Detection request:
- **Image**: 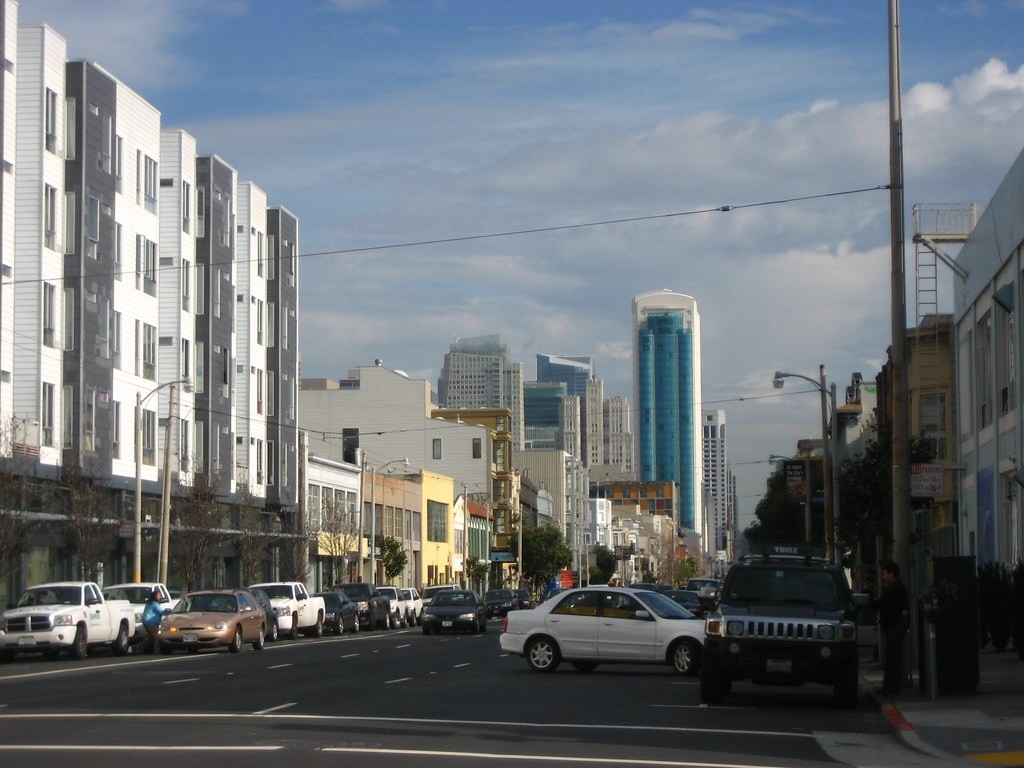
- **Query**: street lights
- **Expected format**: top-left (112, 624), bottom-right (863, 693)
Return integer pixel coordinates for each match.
top-left (773, 369), bottom-right (843, 569)
top-left (132, 376), bottom-right (196, 603)
top-left (768, 451), bottom-right (813, 561)
top-left (605, 516), bottom-right (632, 548)
top-left (368, 457), bottom-right (412, 585)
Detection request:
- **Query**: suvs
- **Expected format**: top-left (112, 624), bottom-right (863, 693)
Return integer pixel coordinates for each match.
top-left (156, 589), bottom-right (267, 656)
top-left (375, 586), bottom-right (410, 629)
top-left (700, 550), bottom-right (872, 703)
top-left (401, 587), bottom-right (422, 627)
top-left (328, 581), bottom-right (392, 632)
top-left (420, 584), bottom-right (469, 605)
top-left (422, 589), bottom-right (487, 636)
top-left (234, 588), bottom-right (279, 642)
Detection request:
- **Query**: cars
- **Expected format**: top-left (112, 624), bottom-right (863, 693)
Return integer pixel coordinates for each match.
top-left (500, 587), bottom-right (707, 674)
top-left (482, 577), bottom-right (724, 618)
top-left (309, 592), bottom-right (360, 636)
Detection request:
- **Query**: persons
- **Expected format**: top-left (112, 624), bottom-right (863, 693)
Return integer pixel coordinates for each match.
top-left (874, 562), bottom-right (913, 694)
top-left (141, 591), bottom-right (168, 655)
top-left (860, 575), bottom-right (876, 596)
top-left (461, 594), bottom-right (470, 603)
top-left (512, 595), bottom-right (521, 610)
top-left (224, 600), bottom-right (237, 613)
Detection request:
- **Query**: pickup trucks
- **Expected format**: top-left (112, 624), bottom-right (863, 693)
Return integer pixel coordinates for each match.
top-left (248, 580), bottom-right (326, 641)
top-left (101, 582), bottom-right (183, 643)
top-left (0, 581), bottom-right (137, 661)
